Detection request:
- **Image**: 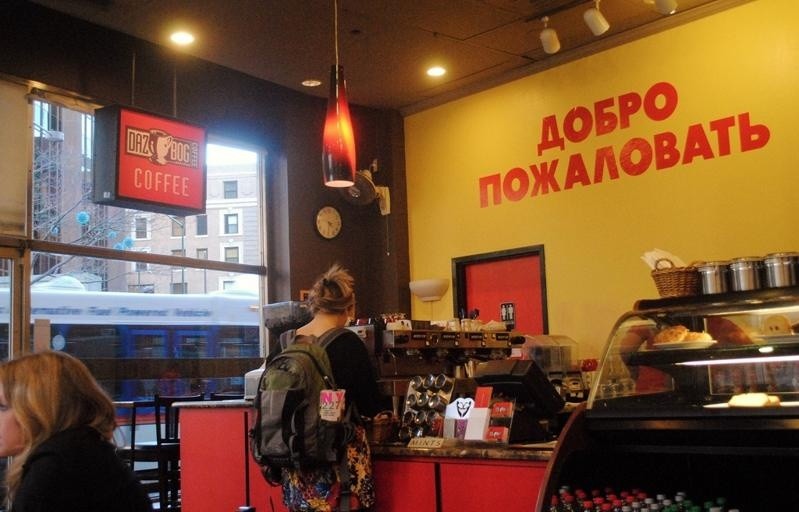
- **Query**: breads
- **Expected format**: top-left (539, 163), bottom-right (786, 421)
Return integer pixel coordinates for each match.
top-left (654, 325), bottom-right (704, 343)
top-left (728, 393), bottom-right (780, 407)
top-left (764, 314), bottom-right (791, 335)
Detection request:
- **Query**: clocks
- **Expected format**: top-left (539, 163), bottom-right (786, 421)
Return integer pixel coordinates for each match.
top-left (313, 205), bottom-right (344, 238)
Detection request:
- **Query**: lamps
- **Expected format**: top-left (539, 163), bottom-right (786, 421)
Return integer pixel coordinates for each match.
top-left (527, 1), bottom-right (681, 56)
top-left (320, 0), bottom-right (357, 187)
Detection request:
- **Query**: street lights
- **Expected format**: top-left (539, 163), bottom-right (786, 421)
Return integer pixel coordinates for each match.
top-left (33, 121), bottom-right (64, 144)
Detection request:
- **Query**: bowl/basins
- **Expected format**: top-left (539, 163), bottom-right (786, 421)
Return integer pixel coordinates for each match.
top-left (408, 277), bottom-right (450, 303)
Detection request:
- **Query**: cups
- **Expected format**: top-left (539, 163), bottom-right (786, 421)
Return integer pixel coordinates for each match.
top-left (399, 372), bottom-right (452, 441)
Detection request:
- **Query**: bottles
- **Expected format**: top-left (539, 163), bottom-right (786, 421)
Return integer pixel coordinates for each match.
top-left (550, 485), bottom-right (750, 512)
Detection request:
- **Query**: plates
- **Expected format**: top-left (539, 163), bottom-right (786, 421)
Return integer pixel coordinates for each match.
top-left (651, 340), bottom-right (718, 351)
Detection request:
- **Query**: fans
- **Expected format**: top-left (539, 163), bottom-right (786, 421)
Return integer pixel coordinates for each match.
top-left (333, 160), bottom-right (393, 219)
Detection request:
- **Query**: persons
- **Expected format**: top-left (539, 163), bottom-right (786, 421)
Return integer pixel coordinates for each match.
top-left (1, 350), bottom-right (155, 512)
top-left (264, 264), bottom-right (391, 511)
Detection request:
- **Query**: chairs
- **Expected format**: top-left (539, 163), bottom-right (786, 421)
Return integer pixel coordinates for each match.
top-left (208, 391), bottom-right (245, 403)
top-left (119, 400), bottom-right (177, 511)
top-left (154, 388), bottom-right (207, 511)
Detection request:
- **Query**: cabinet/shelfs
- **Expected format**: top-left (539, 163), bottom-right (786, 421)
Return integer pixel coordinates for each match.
top-left (536, 289), bottom-right (798, 510)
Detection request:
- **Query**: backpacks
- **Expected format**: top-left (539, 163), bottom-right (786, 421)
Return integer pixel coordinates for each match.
top-left (249, 327), bottom-right (355, 463)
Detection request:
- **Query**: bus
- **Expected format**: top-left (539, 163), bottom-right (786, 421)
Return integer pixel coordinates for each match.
top-left (1, 274), bottom-right (260, 485)
